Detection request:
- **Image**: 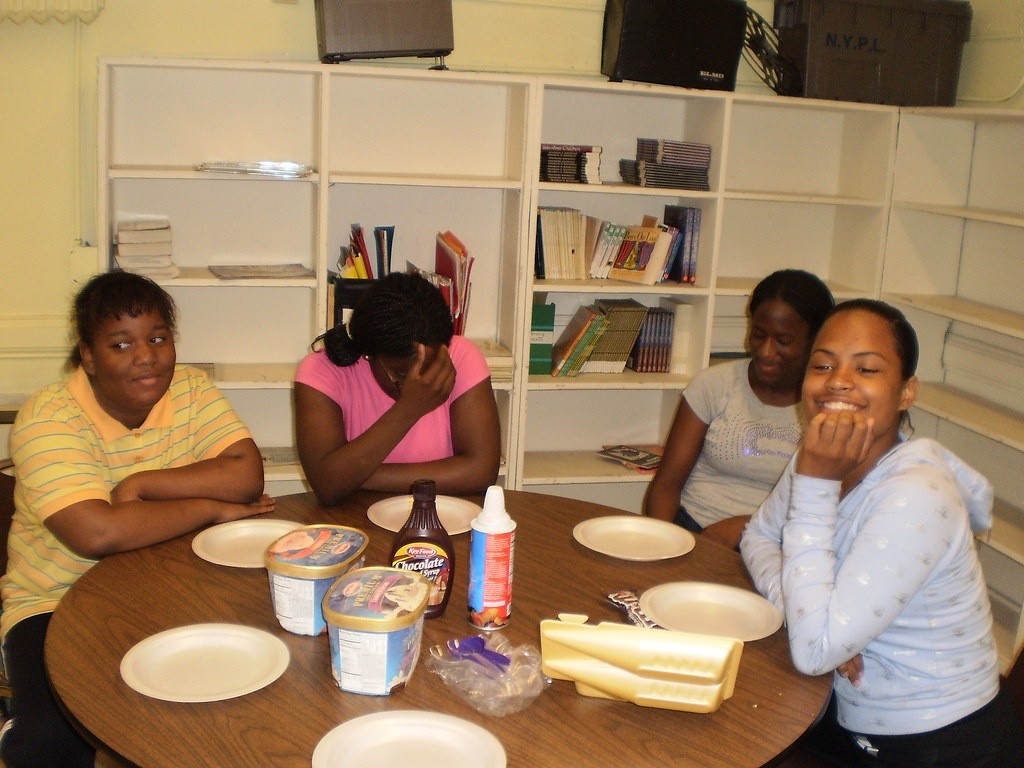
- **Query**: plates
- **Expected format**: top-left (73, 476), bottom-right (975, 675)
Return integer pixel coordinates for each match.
top-left (638, 581), bottom-right (784, 643)
top-left (572, 516), bottom-right (696, 562)
top-left (312, 710), bottom-right (508, 768)
top-left (120, 622), bottom-right (291, 703)
top-left (367, 494), bottom-right (483, 536)
top-left (192, 518), bottom-right (307, 568)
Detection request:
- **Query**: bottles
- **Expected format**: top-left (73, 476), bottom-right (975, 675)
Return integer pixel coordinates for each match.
top-left (388, 478), bottom-right (456, 620)
top-left (466, 485), bottom-right (518, 630)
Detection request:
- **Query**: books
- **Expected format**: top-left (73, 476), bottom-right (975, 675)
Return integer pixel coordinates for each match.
top-left (208, 264), bottom-right (312, 279)
top-left (593, 444), bottom-right (665, 475)
top-left (529, 296), bottom-right (693, 378)
top-left (405, 230), bottom-right (475, 336)
top-left (466, 337), bottom-right (513, 382)
top-left (535, 205), bottom-right (701, 286)
top-left (539, 143), bottom-right (603, 184)
top-left (618, 137), bottom-right (711, 191)
top-left (327, 223), bottom-right (396, 330)
top-left (112, 216), bottom-right (180, 279)
top-left (255, 446), bottom-right (303, 466)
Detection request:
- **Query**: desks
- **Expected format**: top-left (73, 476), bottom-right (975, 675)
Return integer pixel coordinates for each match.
top-left (43, 480), bottom-right (834, 768)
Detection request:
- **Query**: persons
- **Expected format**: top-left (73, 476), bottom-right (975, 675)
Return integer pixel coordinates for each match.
top-left (640, 270), bottom-right (1024, 768)
top-left (0, 271), bottom-right (275, 768)
top-left (292, 272), bottom-right (501, 505)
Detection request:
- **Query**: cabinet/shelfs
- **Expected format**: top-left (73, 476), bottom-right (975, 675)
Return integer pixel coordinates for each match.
top-left (92, 60), bottom-right (1024, 678)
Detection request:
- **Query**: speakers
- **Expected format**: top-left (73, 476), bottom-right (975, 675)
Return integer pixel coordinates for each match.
top-left (601, 0), bottom-right (748, 92)
top-left (315, 0), bottom-right (455, 71)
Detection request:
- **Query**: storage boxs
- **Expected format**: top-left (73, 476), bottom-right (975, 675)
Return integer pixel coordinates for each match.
top-left (775, 1), bottom-right (973, 106)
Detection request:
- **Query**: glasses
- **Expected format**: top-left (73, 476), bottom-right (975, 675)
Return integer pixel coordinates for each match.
top-left (375, 354), bottom-right (404, 390)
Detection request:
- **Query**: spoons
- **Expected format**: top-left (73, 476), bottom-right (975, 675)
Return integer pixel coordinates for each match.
top-left (429, 636), bottom-right (511, 684)
